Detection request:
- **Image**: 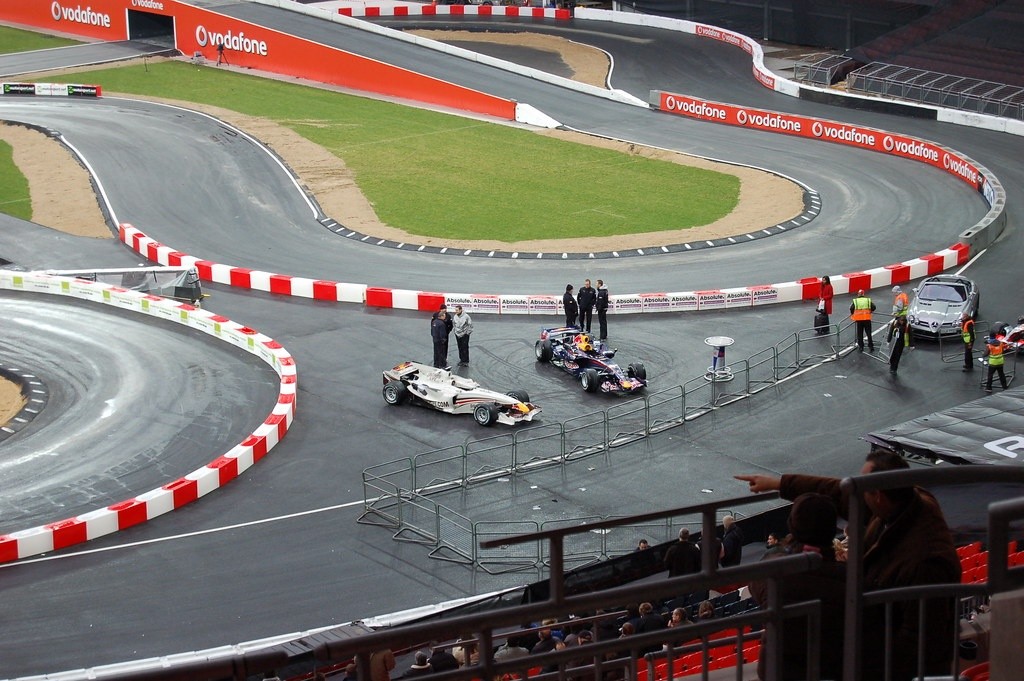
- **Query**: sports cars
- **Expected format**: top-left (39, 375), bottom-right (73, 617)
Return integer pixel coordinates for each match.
top-left (906, 274), bottom-right (980, 341)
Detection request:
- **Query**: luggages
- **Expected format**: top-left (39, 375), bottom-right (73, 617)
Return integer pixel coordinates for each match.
top-left (814, 308), bottom-right (827, 335)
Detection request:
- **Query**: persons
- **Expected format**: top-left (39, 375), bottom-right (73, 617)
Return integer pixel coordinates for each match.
top-left (850, 291), bottom-right (877, 352)
top-left (733, 453), bottom-right (962, 681)
top-left (818, 276), bottom-right (834, 334)
top-left (892, 286), bottom-right (909, 319)
top-left (982, 332), bottom-right (1007, 392)
top-left (887, 315), bottom-right (915, 375)
top-left (563, 279), bottom-right (609, 341)
top-left (961, 313), bottom-right (977, 371)
top-left (343, 513), bottom-right (741, 681)
top-left (431, 304), bottom-right (473, 370)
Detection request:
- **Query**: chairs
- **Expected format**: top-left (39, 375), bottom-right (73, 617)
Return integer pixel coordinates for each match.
top-left (472, 541), bottom-right (1024, 681)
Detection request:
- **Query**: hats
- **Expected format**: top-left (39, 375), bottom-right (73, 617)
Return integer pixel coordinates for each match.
top-left (892, 286), bottom-right (902, 295)
top-left (574, 631), bottom-right (592, 640)
top-left (566, 284), bottom-right (573, 291)
top-left (858, 289), bottom-right (864, 295)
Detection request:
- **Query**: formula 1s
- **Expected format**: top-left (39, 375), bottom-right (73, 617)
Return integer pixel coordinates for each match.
top-left (380, 359), bottom-right (543, 429)
top-left (533, 325), bottom-right (648, 398)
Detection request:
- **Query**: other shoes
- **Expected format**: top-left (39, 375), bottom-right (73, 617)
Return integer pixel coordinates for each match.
top-left (890, 368), bottom-right (896, 375)
top-left (598, 338), bottom-right (606, 341)
top-left (859, 347), bottom-right (863, 350)
top-left (868, 344), bottom-right (874, 351)
top-left (962, 365), bottom-right (972, 372)
top-left (458, 361), bottom-right (463, 365)
top-left (463, 361), bottom-right (469, 366)
top-left (985, 388), bottom-right (992, 392)
